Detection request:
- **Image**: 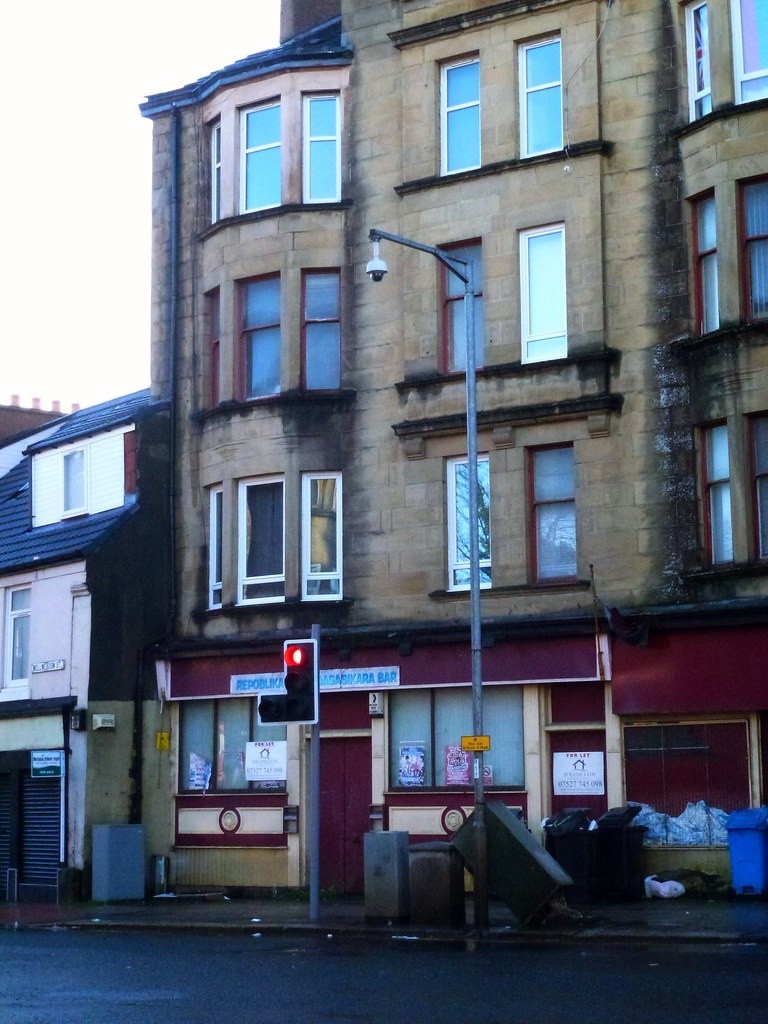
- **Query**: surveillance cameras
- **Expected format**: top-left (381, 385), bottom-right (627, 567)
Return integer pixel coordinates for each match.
top-left (365, 259), bottom-right (389, 282)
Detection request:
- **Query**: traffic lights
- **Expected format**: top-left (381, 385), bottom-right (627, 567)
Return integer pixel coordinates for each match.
top-left (256, 639), bottom-right (320, 724)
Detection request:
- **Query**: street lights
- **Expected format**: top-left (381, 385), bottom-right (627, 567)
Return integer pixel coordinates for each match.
top-left (366, 228), bottom-right (490, 959)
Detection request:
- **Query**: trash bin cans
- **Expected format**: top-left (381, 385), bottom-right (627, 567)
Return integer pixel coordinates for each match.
top-left (725, 807), bottom-right (768, 896)
top-left (543, 805), bottom-right (649, 903)
